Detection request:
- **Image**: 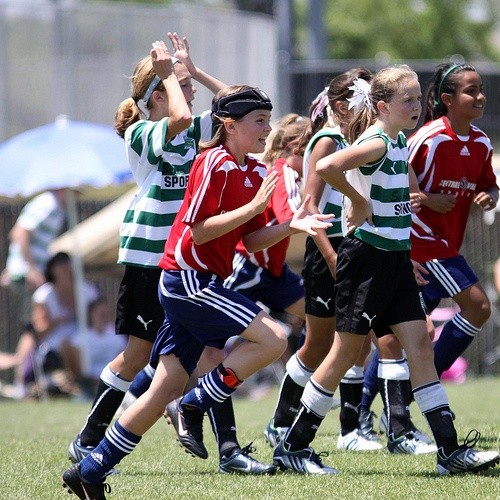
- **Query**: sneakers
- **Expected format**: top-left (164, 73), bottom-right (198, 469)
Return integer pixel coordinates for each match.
top-left (62, 463), bottom-right (113, 500)
top-left (164, 396), bottom-right (208, 459)
top-left (68, 434), bottom-right (119, 474)
top-left (359, 411), bottom-right (378, 441)
top-left (385, 431), bottom-right (439, 454)
top-left (437, 429), bottom-right (500, 476)
top-left (379, 412), bottom-right (432, 444)
top-left (337, 429), bottom-right (383, 451)
top-left (273, 438), bottom-right (339, 476)
top-left (219, 442), bottom-right (277, 475)
top-left (264, 417), bottom-right (290, 448)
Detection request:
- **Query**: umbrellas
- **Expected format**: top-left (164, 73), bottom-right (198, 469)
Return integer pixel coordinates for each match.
top-left (0, 114), bottom-right (136, 378)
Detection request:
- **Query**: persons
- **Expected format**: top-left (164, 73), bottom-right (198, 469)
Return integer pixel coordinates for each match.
top-left (270, 64), bottom-right (500, 476)
top-left (68, 31), bottom-right (277, 476)
top-left (0, 69), bottom-right (500, 455)
top-left (62, 86), bottom-right (335, 500)
top-left (358, 62), bottom-right (500, 444)
